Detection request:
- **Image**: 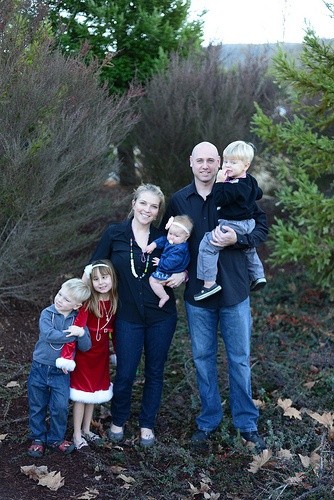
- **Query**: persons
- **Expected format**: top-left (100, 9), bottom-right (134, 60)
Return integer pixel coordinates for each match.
top-left (159, 141), bottom-right (268, 450)
top-left (91, 184), bottom-right (188, 446)
top-left (27, 278), bottom-right (92, 457)
top-left (194, 139), bottom-right (267, 302)
top-left (56, 259), bottom-right (120, 451)
top-left (145, 215), bottom-right (193, 308)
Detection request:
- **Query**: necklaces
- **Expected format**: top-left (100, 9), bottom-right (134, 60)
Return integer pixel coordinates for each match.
top-left (96, 299), bottom-right (113, 341)
top-left (49, 312), bottom-right (75, 351)
top-left (130, 238), bottom-right (151, 279)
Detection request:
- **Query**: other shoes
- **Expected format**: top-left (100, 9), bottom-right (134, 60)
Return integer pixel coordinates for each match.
top-left (193, 282), bottom-right (222, 301)
top-left (28, 444), bottom-right (43, 458)
top-left (108, 422), bottom-right (124, 442)
top-left (243, 432), bottom-right (267, 450)
top-left (250, 278), bottom-right (267, 292)
top-left (50, 440), bottom-right (75, 456)
top-left (71, 437), bottom-right (91, 454)
top-left (80, 430), bottom-right (101, 444)
top-left (139, 428), bottom-right (155, 448)
top-left (190, 430), bottom-right (209, 442)
